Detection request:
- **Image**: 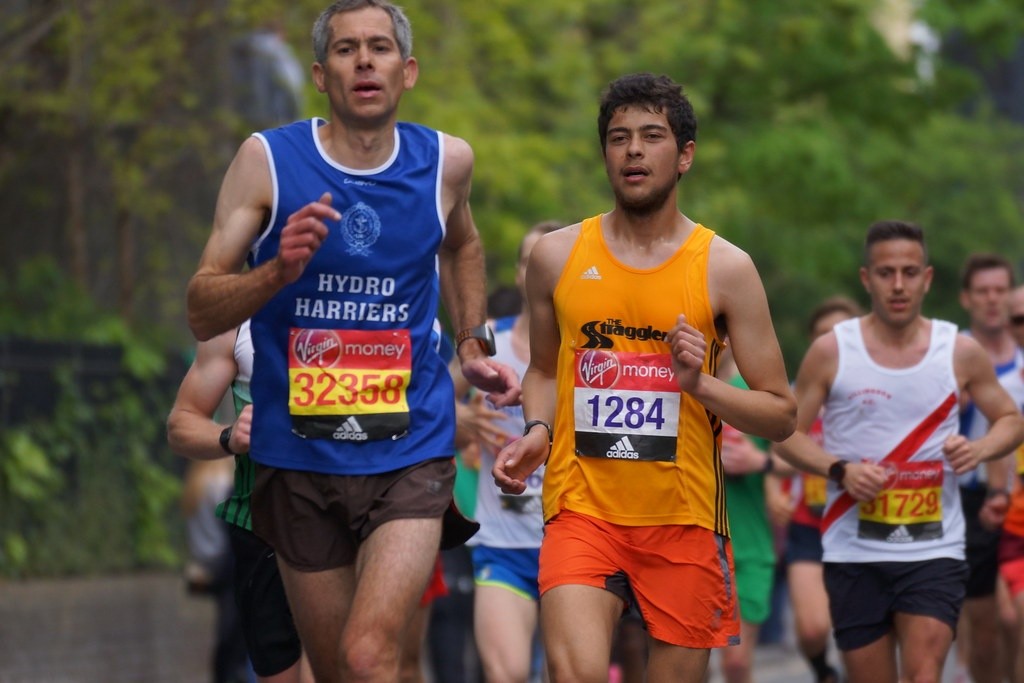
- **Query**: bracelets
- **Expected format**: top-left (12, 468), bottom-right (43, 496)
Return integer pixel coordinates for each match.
top-left (525, 420), bottom-right (551, 435)
top-left (985, 488), bottom-right (1010, 497)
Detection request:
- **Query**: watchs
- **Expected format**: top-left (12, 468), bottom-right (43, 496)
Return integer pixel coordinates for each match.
top-left (829, 459), bottom-right (848, 486)
top-left (220, 426), bottom-right (235, 455)
top-left (454, 324), bottom-right (496, 355)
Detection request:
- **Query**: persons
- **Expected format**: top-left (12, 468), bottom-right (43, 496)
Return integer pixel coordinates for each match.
top-left (447, 222), bottom-right (573, 683)
top-left (721, 334), bottom-right (776, 683)
top-left (765, 297), bottom-right (862, 683)
top-left (165, 318), bottom-right (303, 683)
top-left (773, 219), bottom-right (1024, 683)
top-left (185, 0), bottom-right (524, 683)
top-left (958, 255), bottom-right (1024, 683)
top-left (493, 73), bottom-right (798, 683)
